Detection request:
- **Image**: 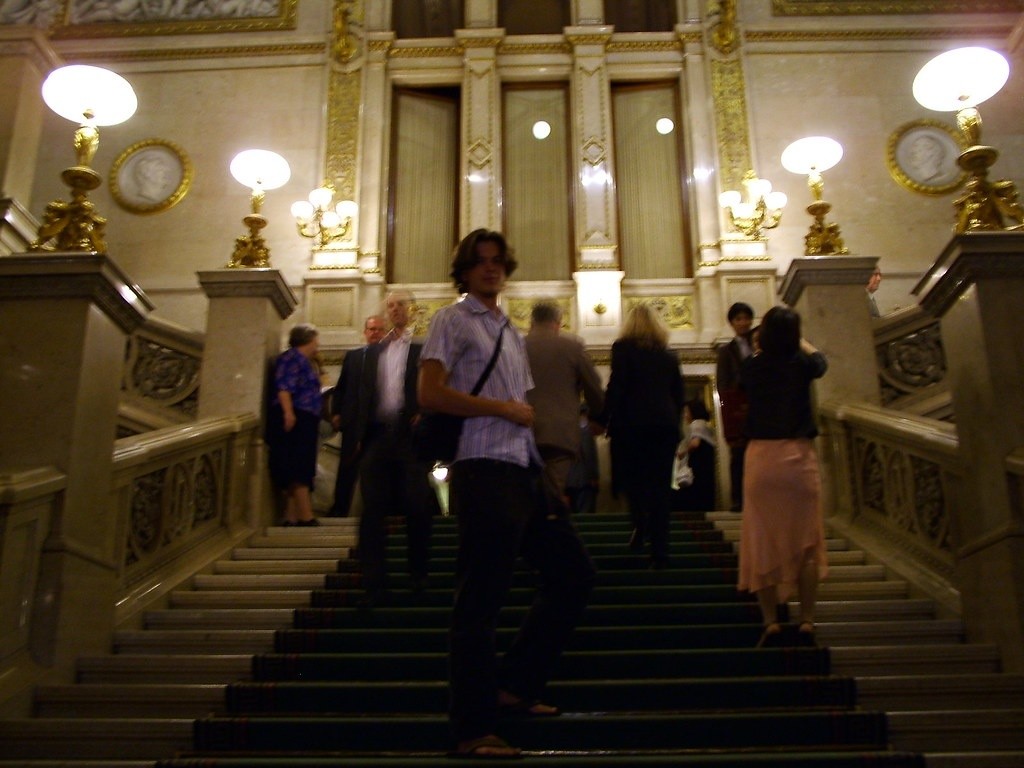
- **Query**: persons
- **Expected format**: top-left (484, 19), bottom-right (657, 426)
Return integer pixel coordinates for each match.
top-left (414, 230), bottom-right (595, 757)
top-left (264, 325), bottom-right (333, 527)
top-left (353, 289), bottom-right (447, 606)
top-left (735, 305), bottom-right (829, 648)
top-left (592, 304), bottom-right (686, 550)
top-left (676, 399), bottom-right (714, 512)
top-left (320, 316), bottom-right (388, 517)
top-left (716, 302), bottom-right (753, 514)
top-left (867, 266), bottom-right (884, 368)
top-left (522, 299), bottom-right (605, 510)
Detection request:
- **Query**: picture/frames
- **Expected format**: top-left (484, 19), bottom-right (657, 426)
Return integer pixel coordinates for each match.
top-left (108, 137), bottom-right (193, 216)
top-left (46, 0), bottom-right (301, 39)
top-left (887, 118), bottom-right (973, 198)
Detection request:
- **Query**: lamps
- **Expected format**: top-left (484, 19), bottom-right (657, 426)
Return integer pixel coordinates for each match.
top-left (37, 65), bottom-right (137, 253)
top-left (719, 170), bottom-right (789, 240)
top-left (912, 46), bottom-right (1024, 231)
top-left (292, 187), bottom-right (356, 249)
top-left (225, 148), bottom-right (290, 269)
top-left (783, 136), bottom-right (849, 254)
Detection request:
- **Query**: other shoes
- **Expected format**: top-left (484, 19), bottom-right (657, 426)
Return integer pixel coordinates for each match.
top-left (653, 560), bottom-right (664, 570)
top-left (321, 507), bottom-right (335, 517)
top-left (352, 589), bottom-right (391, 607)
top-left (627, 527), bottom-right (643, 554)
top-left (283, 521), bottom-right (296, 526)
top-left (412, 577), bottom-right (426, 591)
top-left (730, 504), bottom-right (741, 512)
top-left (296, 519), bottom-right (318, 526)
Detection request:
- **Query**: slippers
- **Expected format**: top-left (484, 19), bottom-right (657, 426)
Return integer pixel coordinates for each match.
top-left (495, 701), bottom-right (561, 717)
top-left (447, 737), bottom-right (521, 759)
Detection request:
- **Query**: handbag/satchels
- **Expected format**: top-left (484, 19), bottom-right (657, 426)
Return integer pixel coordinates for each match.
top-left (411, 412), bottom-right (463, 465)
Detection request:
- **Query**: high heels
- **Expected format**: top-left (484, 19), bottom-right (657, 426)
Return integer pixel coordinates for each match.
top-left (798, 621), bottom-right (818, 647)
top-left (756, 621), bottom-right (785, 648)
